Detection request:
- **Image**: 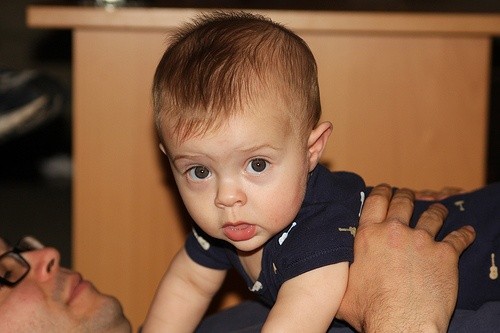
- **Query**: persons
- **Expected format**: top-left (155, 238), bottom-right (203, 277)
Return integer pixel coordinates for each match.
top-left (139, 8), bottom-right (500, 332)
top-left (1, 182), bottom-right (477, 333)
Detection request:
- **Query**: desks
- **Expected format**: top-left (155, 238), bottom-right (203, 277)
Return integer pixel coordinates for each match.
top-left (27, 5), bottom-right (500, 333)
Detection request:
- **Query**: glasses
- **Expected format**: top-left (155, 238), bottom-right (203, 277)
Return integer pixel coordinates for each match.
top-left (0, 232), bottom-right (47, 288)
